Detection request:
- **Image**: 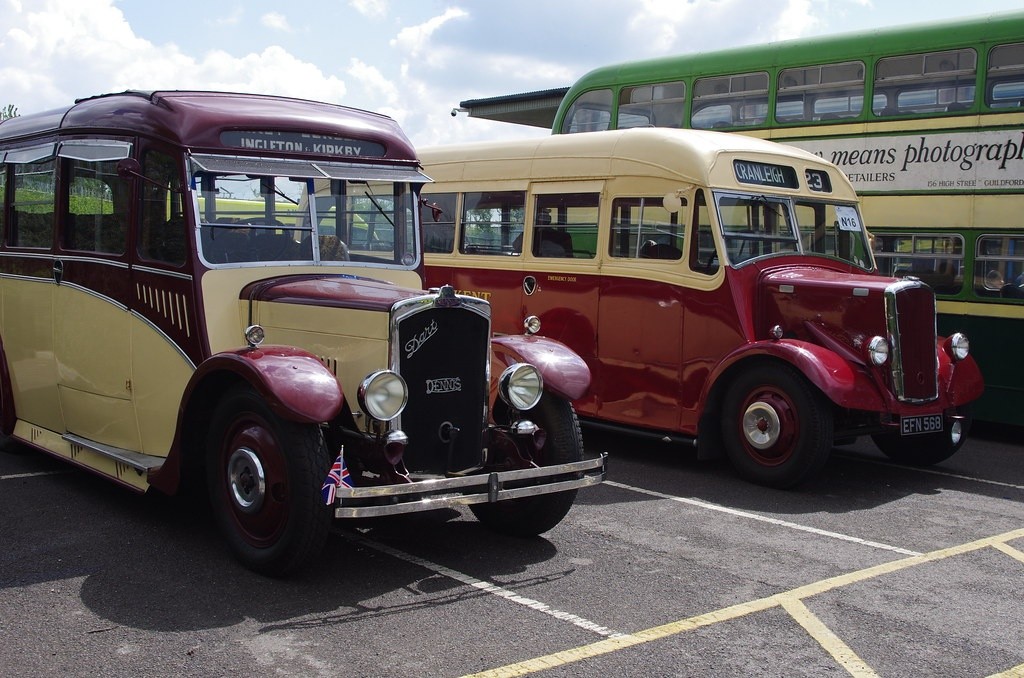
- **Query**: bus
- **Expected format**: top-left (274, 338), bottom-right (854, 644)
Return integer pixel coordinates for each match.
top-left (279, 128), bottom-right (985, 487)
top-left (3, 86), bottom-right (611, 575)
top-left (550, 8), bottom-right (1024, 435)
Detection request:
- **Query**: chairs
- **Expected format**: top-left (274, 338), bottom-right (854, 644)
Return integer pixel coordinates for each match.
top-left (517, 223), bottom-right (758, 275)
top-left (13, 209), bottom-right (313, 255)
